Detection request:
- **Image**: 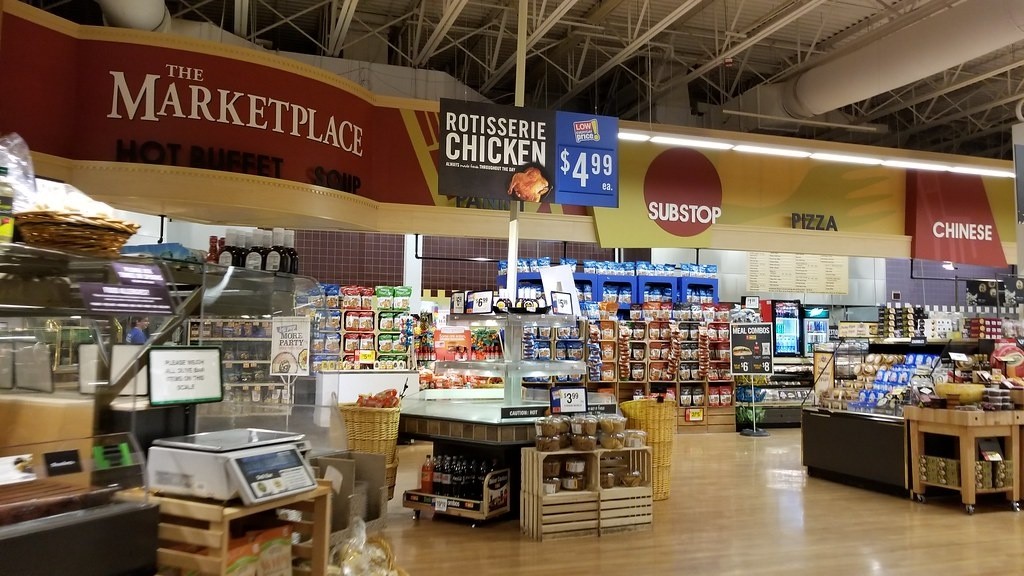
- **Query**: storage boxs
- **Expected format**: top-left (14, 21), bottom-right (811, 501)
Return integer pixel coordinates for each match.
top-left (878, 307), bottom-right (1002, 340)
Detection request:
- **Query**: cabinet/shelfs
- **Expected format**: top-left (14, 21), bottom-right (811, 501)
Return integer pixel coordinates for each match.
top-left (184, 272), bottom-right (737, 433)
top-left (519, 447), bottom-right (654, 542)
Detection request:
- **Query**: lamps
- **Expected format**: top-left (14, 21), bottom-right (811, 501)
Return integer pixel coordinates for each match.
top-left (941, 262), bottom-right (954, 271)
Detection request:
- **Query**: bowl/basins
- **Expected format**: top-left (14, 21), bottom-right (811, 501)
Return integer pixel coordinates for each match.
top-left (536, 413), bottom-right (646, 495)
top-left (953, 388), bottom-right (1014, 412)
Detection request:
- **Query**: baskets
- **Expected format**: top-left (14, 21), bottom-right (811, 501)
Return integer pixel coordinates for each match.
top-left (386, 461), bottom-right (399, 501)
top-left (339, 402), bottom-right (401, 465)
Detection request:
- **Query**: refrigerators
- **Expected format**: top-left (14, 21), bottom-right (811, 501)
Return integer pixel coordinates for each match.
top-left (797, 303), bottom-right (830, 358)
top-left (762, 299), bottom-right (800, 356)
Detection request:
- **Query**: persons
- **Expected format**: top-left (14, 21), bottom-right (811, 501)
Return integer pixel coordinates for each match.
top-left (125, 314), bottom-right (150, 346)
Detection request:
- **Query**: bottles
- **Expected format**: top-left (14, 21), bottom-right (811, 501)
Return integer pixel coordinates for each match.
top-left (754, 342), bottom-right (760, 355)
top-left (489, 490), bottom-right (509, 509)
top-left (0, 167), bottom-right (15, 245)
top-left (421, 455), bottom-right (500, 503)
top-left (206, 226), bottom-right (300, 274)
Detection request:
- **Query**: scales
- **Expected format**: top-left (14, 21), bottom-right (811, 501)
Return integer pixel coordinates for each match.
top-left (147, 425), bottom-right (319, 510)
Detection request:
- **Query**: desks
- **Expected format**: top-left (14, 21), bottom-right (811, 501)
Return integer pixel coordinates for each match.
top-left (114, 480), bottom-right (332, 576)
top-left (903, 405), bottom-right (1024, 514)
